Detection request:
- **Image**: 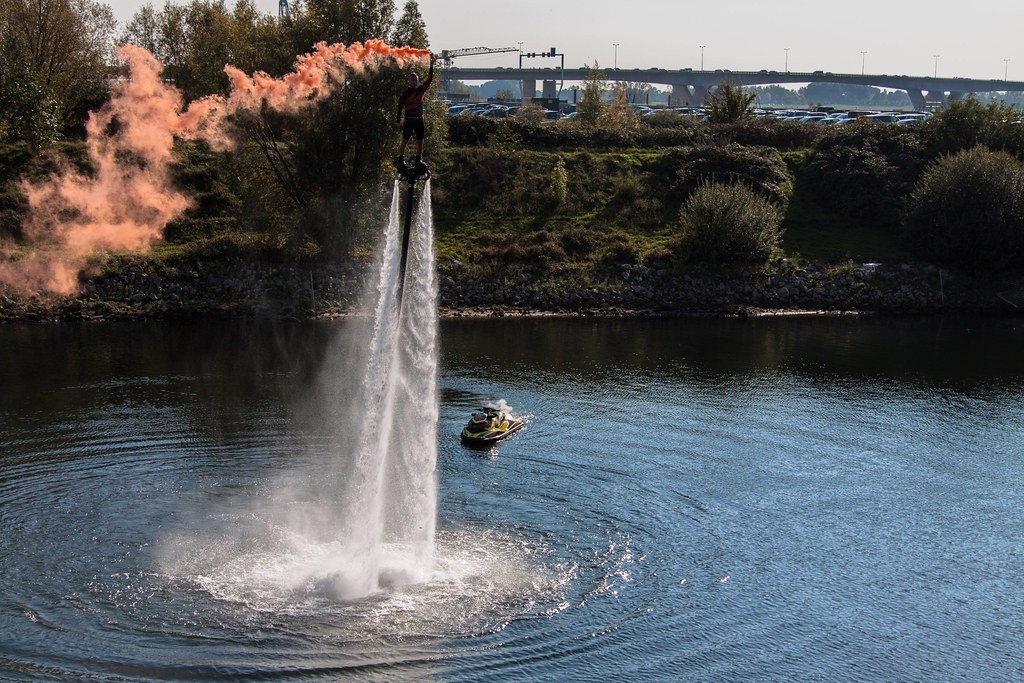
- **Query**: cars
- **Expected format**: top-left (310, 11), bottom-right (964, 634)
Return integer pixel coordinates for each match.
top-left (445, 103), bottom-right (935, 127)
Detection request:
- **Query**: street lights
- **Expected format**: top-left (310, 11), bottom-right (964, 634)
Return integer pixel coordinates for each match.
top-left (933, 54), bottom-right (940, 78)
top-left (1003, 58), bottom-right (1011, 82)
top-left (518, 41), bottom-right (524, 55)
top-left (699, 45), bottom-right (706, 70)
top-left (613, 43), bottom-right (619, 67)
top-left (860, 51), bottom-right (867, 75)
top-left (783, 47), bottom-right (790, 71)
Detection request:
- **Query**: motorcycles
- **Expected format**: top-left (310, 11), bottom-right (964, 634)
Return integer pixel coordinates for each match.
top-left (461, 406), bottom-right (525, 446)
